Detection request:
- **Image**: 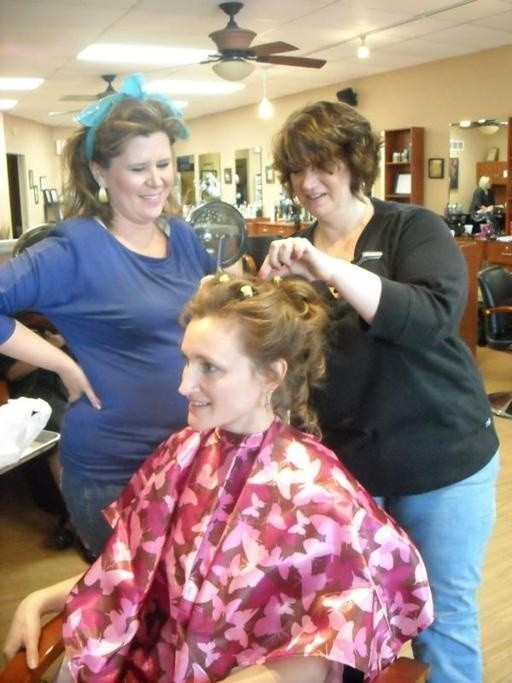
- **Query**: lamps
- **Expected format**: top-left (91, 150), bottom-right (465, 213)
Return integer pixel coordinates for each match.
top-left (355, 33), bottom-right (372, 60)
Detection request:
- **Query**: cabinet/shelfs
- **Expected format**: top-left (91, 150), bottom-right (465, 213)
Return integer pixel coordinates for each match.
top-left (385, 126), bottom-right (424, 205)
top-left (475, 159), bottom-right (509, 237)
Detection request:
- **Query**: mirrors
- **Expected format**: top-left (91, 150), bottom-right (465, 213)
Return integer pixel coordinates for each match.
top-left (446, 121), bottom-right (510, 237)
top-left (176, 145), bottom-right (263, 207)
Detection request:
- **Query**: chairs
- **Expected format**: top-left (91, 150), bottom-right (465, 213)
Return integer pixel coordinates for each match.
top-left (477, 264), bottom-right (512, 419)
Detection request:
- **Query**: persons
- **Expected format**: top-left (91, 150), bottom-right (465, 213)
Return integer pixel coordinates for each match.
top-left (0, 73), bottom-right (219, 558)
top-left (5, 313), bottom-right (76, 490)
top-left (257, 101), bottom-right (500, 683)
top-left (1, 271), bottom-right (435, 683)
top-left (469, 176), bottom-right (492, 233)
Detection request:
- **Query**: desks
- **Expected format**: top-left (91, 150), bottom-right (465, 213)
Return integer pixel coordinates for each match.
top-left (251, 219), bottom-right (298, 236)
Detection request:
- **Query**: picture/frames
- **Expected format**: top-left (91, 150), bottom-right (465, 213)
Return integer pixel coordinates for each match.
top-left (428, 158), bottom-right (443, 178)
top-left (28, 170), bottom-right (58, 204)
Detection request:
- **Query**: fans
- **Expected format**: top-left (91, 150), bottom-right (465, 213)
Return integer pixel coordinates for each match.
top-left (59, 74), bottom-right (118, 102)
top-left (201, 1), bottom-right (327, 83)
